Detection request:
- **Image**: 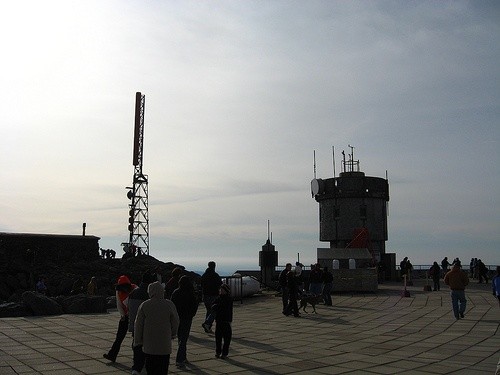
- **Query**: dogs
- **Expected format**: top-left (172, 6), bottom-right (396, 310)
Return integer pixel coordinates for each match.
top-left (297, 293), bottom-right (325, 315)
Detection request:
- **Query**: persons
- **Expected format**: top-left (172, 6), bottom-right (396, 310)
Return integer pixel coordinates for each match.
top-left (87, 276), bottom-right (97, 296)
top-left (441, 256), bottom-right (462, 279)
top-left (492, 266), bottom-right (500, 312)
top-left (400, 257), bottom-right (413, 286)
top-left (103, 261), bottom-right (233, 375)
top-left (134, 281), bottom-right (179, 375)
top-left (444, 263), bottom-right (469, 319)
top-left (429, 262), bottom-right (440, 291)
top-left (279, 262), bottom-right (333, 318)
top-left (469, 257), bottom-right (488, 284)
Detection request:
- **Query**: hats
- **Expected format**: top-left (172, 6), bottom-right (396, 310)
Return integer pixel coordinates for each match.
top-left (118, 275), bottom-right (130, 284)
top-left (220, 283), bottom-right (230, 292)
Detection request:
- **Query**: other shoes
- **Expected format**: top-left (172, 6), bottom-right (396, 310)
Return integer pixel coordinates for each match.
top-left (103, 353), bottom-right (116, 362)
top-left (221, 356), bottom-right (230, 363)
top-left (215, 354), bottom-right (220, 358)
top-left (433, 289), bottom-right (437, 291)
top-left (182, 358), bottom-right (190, 365)
top-left (175, 363), bottom-right (181, 368)
top-left (282, 311), bottom-right (289, 317)
top-left (202, 323), bottom-right (211, 333)
top-left (131, 370), bottom-right (141, 375)
top-left (294, 314), bottom-right (301, 317)
top-left (460, 312), bottom-right (464, 318)
